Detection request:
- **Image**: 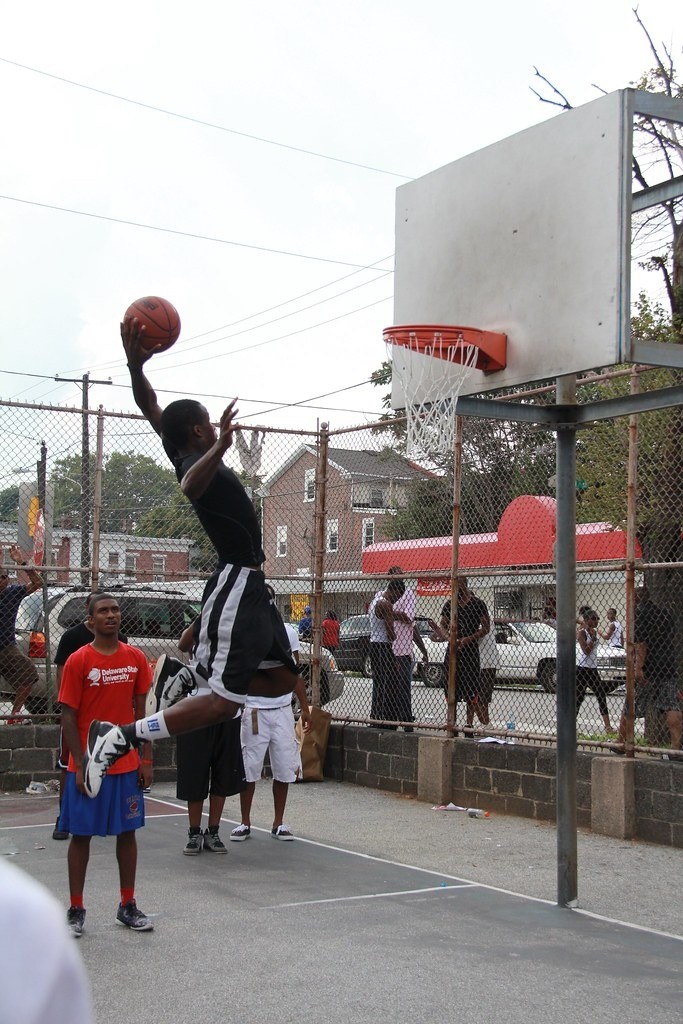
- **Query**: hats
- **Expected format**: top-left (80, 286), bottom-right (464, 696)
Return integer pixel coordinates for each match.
top-left (303, 606), bottom-right (310, 614)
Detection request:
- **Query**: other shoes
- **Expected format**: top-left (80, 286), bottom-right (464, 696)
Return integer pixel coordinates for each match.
top-left (660, 753), bottom-right (681, 763)
top-left (610, 746), bottom-right (626, 754)
top-left (606, 727), bottom-right (618, 734)
top-left (475, 722), bottom-right (494, 738)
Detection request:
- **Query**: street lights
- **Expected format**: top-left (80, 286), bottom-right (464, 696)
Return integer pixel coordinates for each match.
top-left (12, 466), bottom-right (91, 586)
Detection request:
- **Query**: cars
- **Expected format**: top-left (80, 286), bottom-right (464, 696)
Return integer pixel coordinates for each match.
top-left (408, 618), bottom-right (643, 694)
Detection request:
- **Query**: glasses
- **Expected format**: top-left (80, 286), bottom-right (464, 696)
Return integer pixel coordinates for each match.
top-left (587, 617), bottom-right (600, 621)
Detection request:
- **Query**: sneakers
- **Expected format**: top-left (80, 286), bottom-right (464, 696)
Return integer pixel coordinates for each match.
top-left (204, 829), bottom-right (226, 855)
top-left (83, 718), bottom-right (134, 800)
top-left (52, 813), bottom-right (70, 838)
top-left (229, 824), bottom-right (251, 842)
top-left (66, 903), bottom-right (87, 936)
top-left (271, 824), bottom-right (295, 842)
top-left (145, 651), bottom-right (198, 719)
top-left (7, 711), bottom-right (31, 727)
top-left (115, 898), bottom-right (155, 931)
top-left (183, 823), bottom-right (206, 856)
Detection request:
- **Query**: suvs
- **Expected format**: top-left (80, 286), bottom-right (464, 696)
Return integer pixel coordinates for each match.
top-left (0, 584), bottom-right (345, 725)
top-left (331, 613), bottom-right (433, 679)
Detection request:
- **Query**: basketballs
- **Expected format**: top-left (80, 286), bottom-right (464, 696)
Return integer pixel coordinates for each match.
top-left (123, 295), bottom-right (182, 353)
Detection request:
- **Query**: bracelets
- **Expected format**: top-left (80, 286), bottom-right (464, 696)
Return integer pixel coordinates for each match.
top-left (20, 560), bottom-right (29, 566)
top-left (142, 760), bottom-right (153, 765)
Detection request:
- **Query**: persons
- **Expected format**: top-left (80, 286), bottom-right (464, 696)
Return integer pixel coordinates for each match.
top-left (577, 605), bottom-right (591, 629)
top-left (58, 593), bottom-right (154, 937)
top-left (81, 316), bottom-right (271, 800)
top-left (576, 610), bottom-right (617, 734)
top-left (542, 608), bottom-right (556, 628)
top-left (368, 580), bottom-right (405, 731)
top-left (321, 610), bottom-right (340, 655)
top-left (376, 566), bottom-right (428, 732)
top-left (176, 616), bottom-right (248, 856)
top-left (0, 544), bottom-right (46, 725)
top-left (597, 608), bottom-right (624, 648)
top-left (283, 623), bottom-right (300, 666)
top-left (52, 591), bottom-right (129, 841)
top-left (428, 575), bottom-right (490, 738)
top-left (467, 590), bottom-right (501, 728)
top-left (230, 584), bottom-right (315, 841)
top-left (605, 582), bottom-right (683, 761)
top-left (298, 607), bottom-right (313, 643)
